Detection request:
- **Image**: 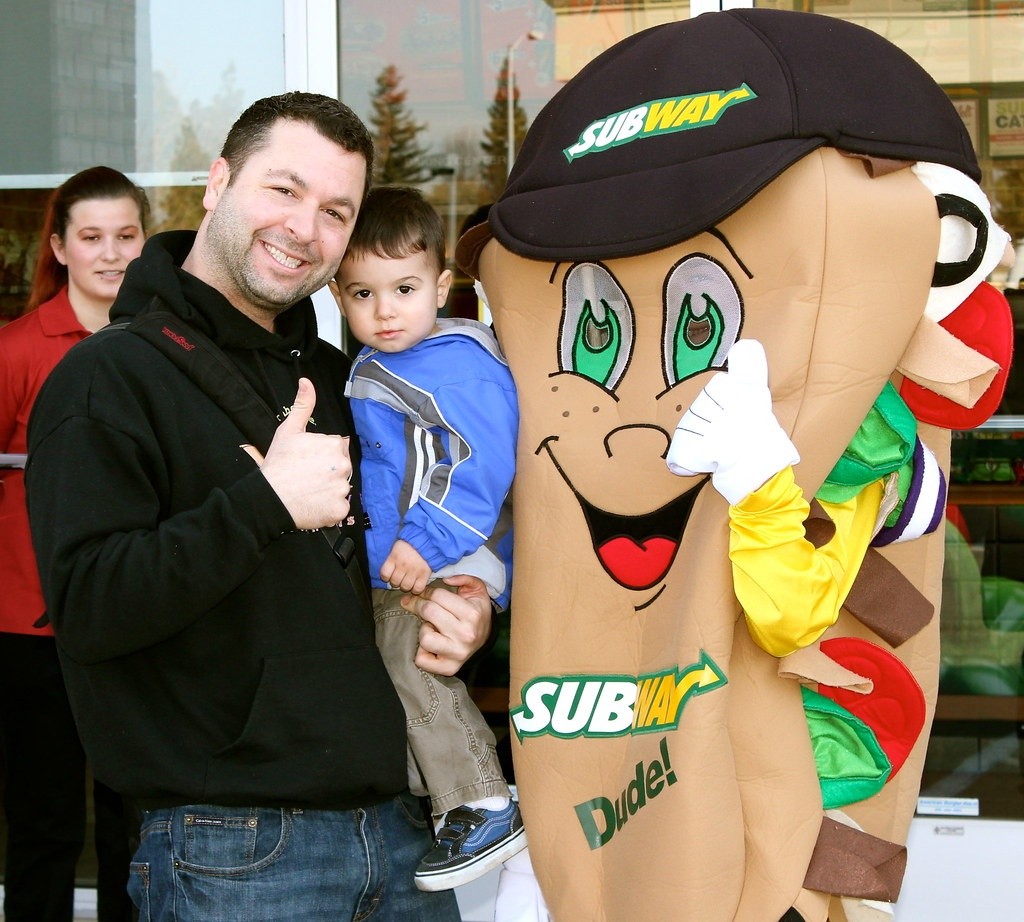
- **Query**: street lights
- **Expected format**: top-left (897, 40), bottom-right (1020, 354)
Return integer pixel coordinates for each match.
top-left (508, 28), bottom-right (542, 176)
top-left (430, 155), bottom-right (459, 267)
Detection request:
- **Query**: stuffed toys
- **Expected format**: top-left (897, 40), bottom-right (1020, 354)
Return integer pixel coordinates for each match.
top-left (454, 11), bottom-right (1012, 921)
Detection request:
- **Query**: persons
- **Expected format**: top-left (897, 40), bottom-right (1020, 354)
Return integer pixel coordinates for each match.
top-left (0, 167), bottom-right (150, 922)
top-left (328, 187), bottom-right (529, 892)
top-left (23, 93), bottom-right (490, 922)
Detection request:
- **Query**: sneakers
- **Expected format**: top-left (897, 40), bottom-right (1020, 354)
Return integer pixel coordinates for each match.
top-left (413, 799), bottom-right (528, 892)
